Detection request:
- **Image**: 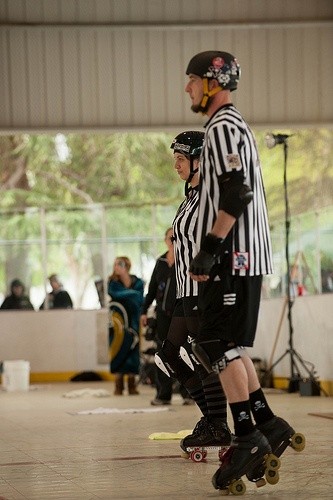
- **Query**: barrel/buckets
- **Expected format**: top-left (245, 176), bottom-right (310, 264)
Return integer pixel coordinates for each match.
top-left (2, 360), bottom-right (30, 392)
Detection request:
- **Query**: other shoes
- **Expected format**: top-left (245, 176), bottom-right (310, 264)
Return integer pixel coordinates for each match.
top-left (151, 398), bottom-right (170, 404)
top-left (182, 399), bottom-right (196, 405)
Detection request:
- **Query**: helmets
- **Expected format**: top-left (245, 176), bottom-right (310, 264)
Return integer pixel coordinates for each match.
top-left (170, 130), bottom-right (206, 157)
top-left (187, 51), bottom-right (241, 92)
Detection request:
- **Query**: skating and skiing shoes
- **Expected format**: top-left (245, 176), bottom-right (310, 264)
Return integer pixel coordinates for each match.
top-left (211, 429), bottom-right (281, 495)
top-left (179, 419), bottom-right (234, 462)
top-left (246, 417), bottom-right (306, 487)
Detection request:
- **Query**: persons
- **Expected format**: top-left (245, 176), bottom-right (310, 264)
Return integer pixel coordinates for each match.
top-left (170, 131), bottom-right (233, 452)
top-left (0, 280), bottom-right (35, 311)
top-left (186, 50), bottom-right (297, 490)
top-left (39, 274), bottom-right (74, 310)
top-left (107, 229), bottom-right (195, 409)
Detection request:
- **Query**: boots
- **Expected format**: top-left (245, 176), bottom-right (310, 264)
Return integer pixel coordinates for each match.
top-left (128, 377), bottom-right (139, 394)
top-left (115, 377), bottom-right (124, 395)
